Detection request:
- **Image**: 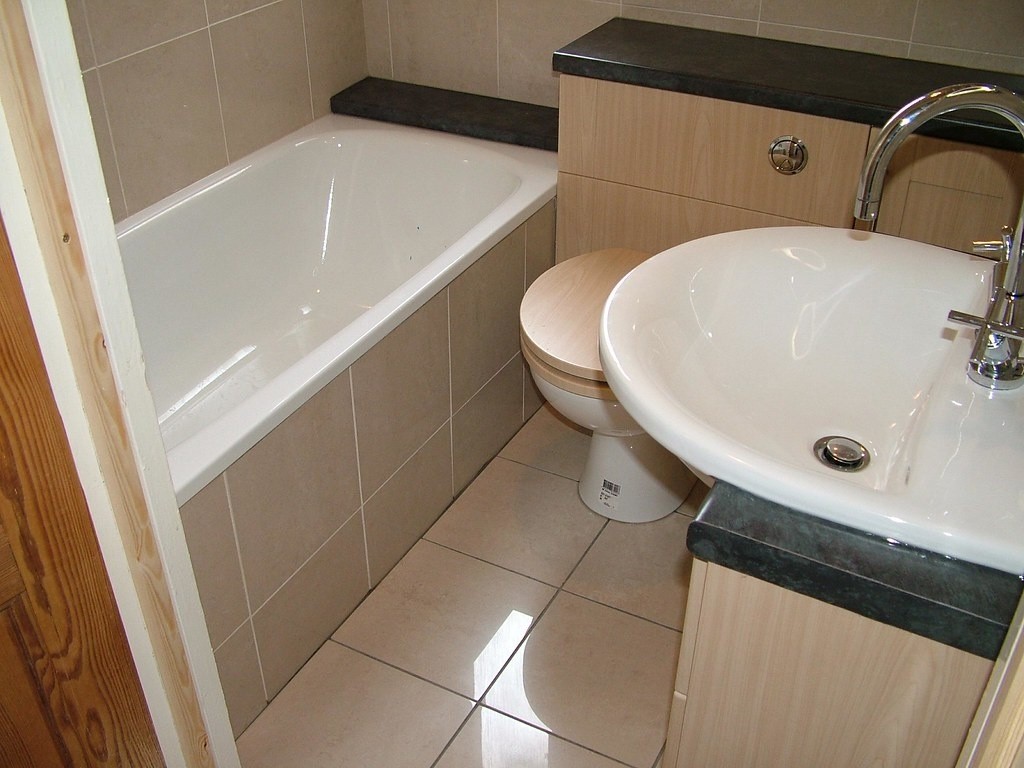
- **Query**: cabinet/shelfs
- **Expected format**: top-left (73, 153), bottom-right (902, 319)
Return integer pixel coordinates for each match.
top-left (553, 72), bottom-right (1024, 267)
top-left (658, 558), bottom-right (999, 768)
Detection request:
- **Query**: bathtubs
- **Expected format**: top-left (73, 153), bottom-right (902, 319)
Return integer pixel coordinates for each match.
top-left (93, 74), bottom-right (560, 741)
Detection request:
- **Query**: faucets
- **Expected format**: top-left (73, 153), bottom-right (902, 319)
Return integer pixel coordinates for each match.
top-left (852, 81), bottom-right (1024, 303)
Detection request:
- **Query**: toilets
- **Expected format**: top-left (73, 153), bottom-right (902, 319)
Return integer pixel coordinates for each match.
top-left (517, 246), bottom-right (699, 525)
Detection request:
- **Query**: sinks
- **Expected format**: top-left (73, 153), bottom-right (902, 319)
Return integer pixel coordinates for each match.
top-left (598, 225), bottom-right (1023, 578)
